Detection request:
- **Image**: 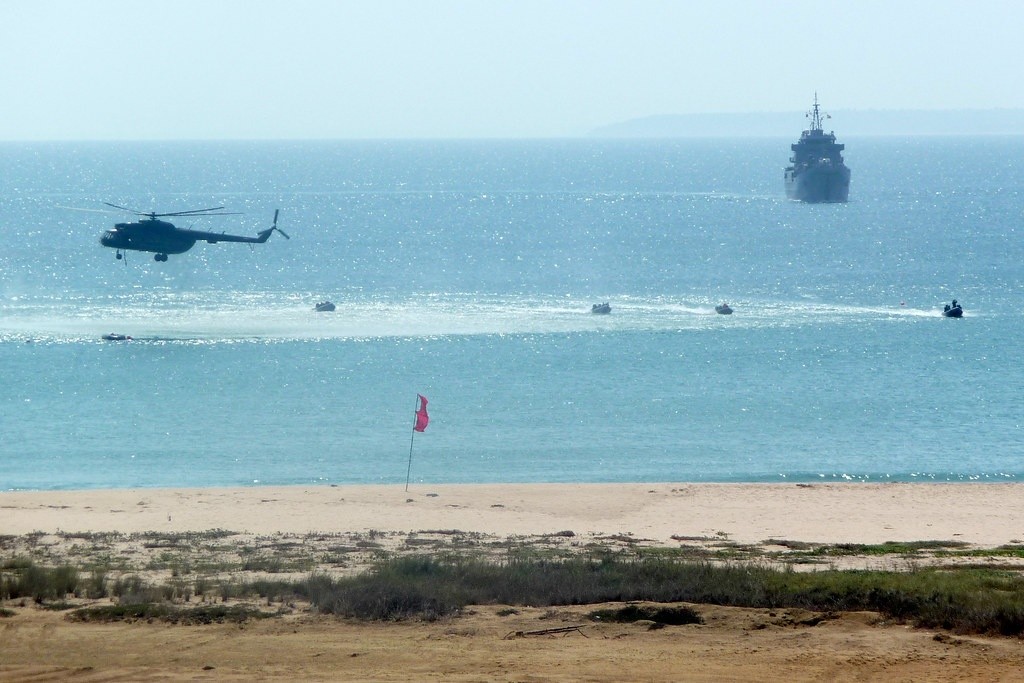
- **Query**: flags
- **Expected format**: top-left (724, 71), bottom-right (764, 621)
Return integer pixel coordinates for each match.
top-left (413, 394), bottom-right (429, 433)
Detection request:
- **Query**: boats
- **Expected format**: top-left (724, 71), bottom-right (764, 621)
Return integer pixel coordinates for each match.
top-left (592, 302), bottom-right (611, 313)
top-left (715, 304), bottom-right (733, 315)
top-left (942, 307), bottom-right (962, 317)
top-left (784, 92), bottom-right (851, 205)
top-left (102, 333), bottom-right (131, 340)
top-left (315, 301), bottom-right (335, 312)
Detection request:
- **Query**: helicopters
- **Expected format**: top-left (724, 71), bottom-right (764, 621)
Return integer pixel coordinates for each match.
top-left (57, 202), bottom-right (289, 262)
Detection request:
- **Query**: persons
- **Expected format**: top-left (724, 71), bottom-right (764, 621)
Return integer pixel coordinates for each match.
top-left (943, 305), bottom-right (950, 312)
top-left (952, 300), bottom-right (962, 308)
